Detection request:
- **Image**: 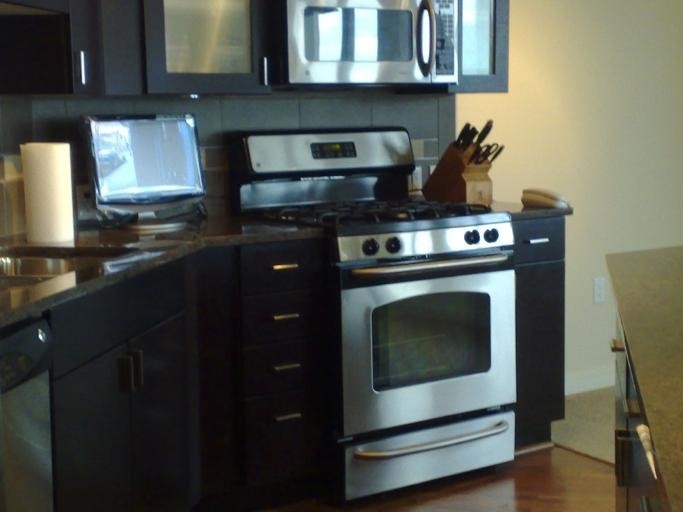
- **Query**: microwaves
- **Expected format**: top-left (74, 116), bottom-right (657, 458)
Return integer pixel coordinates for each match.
top-left (269, 0), bottom-right (459, 91)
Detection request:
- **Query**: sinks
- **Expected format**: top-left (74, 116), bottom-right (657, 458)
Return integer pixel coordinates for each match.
top-left (0, 244), bottom-right (141, 284)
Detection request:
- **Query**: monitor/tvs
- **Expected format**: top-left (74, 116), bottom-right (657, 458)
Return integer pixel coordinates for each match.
top-left (97, 230), bottom-right (195, 279)
top-left (84, 113), bottom-right (207, 229)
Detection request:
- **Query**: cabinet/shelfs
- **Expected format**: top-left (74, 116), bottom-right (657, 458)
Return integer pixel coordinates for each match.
top-left (89, 0), bottom-right (272, 98)
top-left (608, 245), bottom-right (683, 512)
top-left (448, 0), bottom-right (510, 93)
top-left (0, 238), bottom-right (334, 512)
top-left (501, 217), bottom-right (565, 457)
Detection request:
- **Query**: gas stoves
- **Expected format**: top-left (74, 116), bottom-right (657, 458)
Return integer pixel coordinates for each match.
top-left (224, 197), bottom-right (518, 264)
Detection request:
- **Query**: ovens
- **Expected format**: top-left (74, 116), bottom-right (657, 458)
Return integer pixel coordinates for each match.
top-left (338, 247), bottom-right (518, 503)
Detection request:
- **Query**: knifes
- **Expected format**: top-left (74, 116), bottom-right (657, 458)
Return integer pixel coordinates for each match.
top-left (459, 116), bottom-right (504, 166)
